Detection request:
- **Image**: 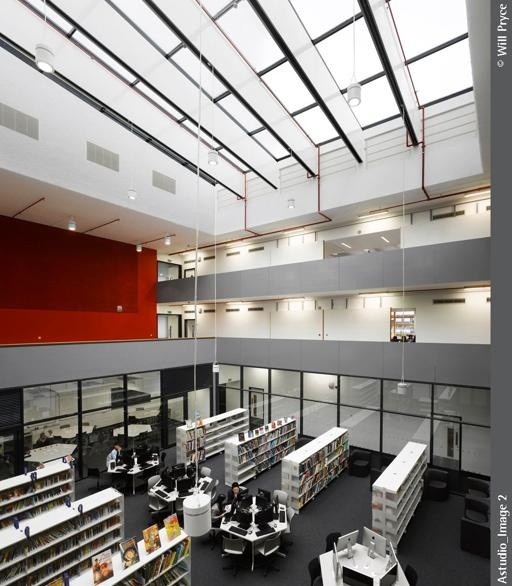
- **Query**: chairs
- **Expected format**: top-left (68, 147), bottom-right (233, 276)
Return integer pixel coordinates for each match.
top-left (423, 467), bottom-right (490, 559)
top-left (221, 530), bottom-right (287, 578)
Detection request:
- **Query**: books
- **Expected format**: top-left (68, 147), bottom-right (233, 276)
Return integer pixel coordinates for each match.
top-left (298, 431), bottom-right (350, 508)
top-left (0, 471), bottom-right (191, 586)
top-left (237, 415), bottom-right (296, 476)
top-left (184, 416), bottom-right (207, 462)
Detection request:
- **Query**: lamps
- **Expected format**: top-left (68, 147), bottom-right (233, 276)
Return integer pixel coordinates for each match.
top-left (395, 100), bottom-right (412, 396)
top-left (181, 0), bottom-right (222, 538)
top-left (35, 0), bottom-right (56, 73)
top-left (346, 1), bottom-right (362, 105)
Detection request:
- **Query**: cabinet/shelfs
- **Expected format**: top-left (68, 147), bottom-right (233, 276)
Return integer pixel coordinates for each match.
top-left (0, 461), bottom-right (191, 585)
top-left (175, 407), bottom-right (428, 556)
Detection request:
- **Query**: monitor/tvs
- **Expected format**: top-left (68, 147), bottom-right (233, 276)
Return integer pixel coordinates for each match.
top-left (336, 530), bottom-right (359, 559)
top-left (379, 563), bottom-right (397, 586)
top-left (224, 487), bottom-right (286, 538)
top-left (110, 442), bottom-right (158, 474)
top-left (362, 527), bottom-right (387, 560)
top-left (332, 542), bottom-right (341, 584)
top-left (341, 565), bottom-right (373, 586)
top-left (153, 464), bottom-right (212, 501)
top-left (385, 541), bottom-right (398, 571)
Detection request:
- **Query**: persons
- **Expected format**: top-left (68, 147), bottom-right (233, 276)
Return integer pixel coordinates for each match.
top-left (229, 482), bottom-right (244, 504)
top-left (37, 433), bottom-right (49, 446)
